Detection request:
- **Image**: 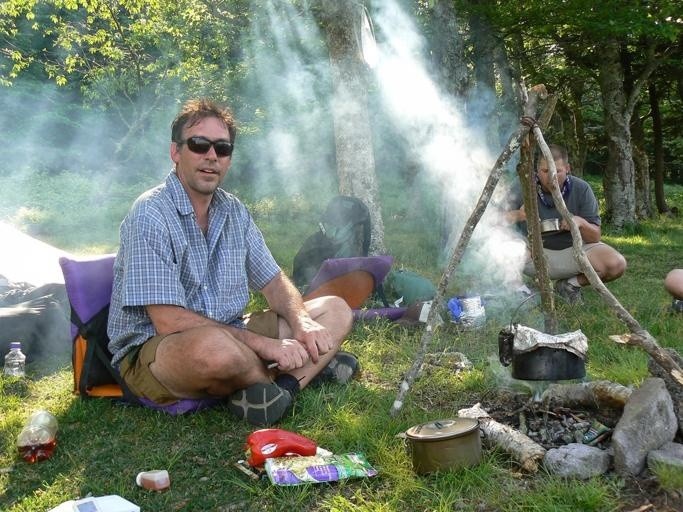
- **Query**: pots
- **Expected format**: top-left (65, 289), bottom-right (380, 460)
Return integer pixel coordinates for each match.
top-left (504, 291), bottom-right (587, 381)
top-left (405, 417), bottom-right (484, 476)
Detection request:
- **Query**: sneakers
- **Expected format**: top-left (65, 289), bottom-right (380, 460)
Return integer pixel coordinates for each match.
top-left (671, 298), bottom-right (683, 312)
top-left (226, 373), bottom-right (300, 426)
top-left (318, 351), bottom-right (359, 380)
top-left (555, 278), bottom-right (584, 307)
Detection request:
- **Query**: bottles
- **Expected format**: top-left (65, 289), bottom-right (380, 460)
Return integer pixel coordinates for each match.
top-left (4, 342), bottom-right (25, 382)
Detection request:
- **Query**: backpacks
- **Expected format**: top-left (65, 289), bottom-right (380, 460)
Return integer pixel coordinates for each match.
top-left (294, 194), bottom-right (370, 284)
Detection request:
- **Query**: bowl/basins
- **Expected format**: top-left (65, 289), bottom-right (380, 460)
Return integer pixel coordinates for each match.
top-left (539, 218), bottom-right (562, 235)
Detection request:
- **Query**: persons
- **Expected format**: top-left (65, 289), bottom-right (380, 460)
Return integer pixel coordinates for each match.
top-left (664, 268), bottom-right (683, 313)
top-left (497, 143), bottom-right (627, 308)
top-left (105, 102), bottom-right (358, 425)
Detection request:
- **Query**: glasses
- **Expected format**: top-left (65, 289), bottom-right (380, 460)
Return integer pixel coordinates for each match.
top-left (177, 136), bottom-right (234, 157)
